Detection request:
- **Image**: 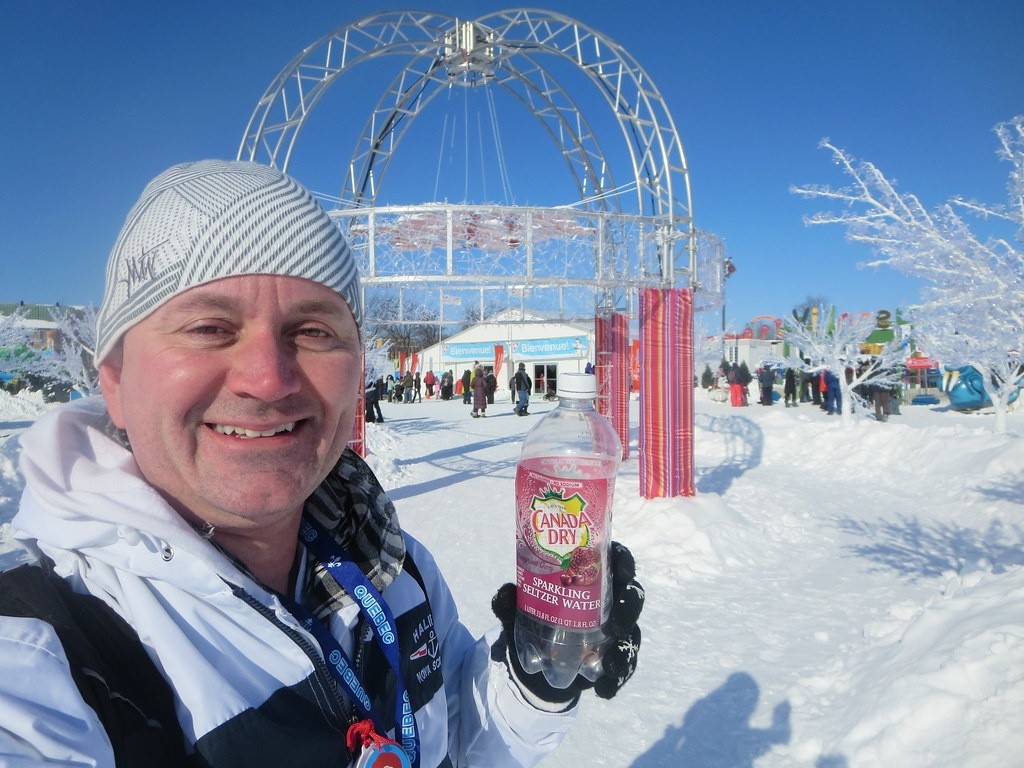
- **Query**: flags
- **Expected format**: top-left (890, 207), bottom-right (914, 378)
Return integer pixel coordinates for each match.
top-left (509, 288), bottom-right (531, 296)
top-left (442, 294), bottom-right (460, 305)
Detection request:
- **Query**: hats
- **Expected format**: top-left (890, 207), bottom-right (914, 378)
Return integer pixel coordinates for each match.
top-left (90, 157), bottom-right (365, 369)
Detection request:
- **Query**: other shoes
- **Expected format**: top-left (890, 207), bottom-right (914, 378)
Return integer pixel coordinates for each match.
top-left (376, 416), bottom-right (384, 424)
top-left (366, 414), bottom-right (376, 423)
top-left (470, 412), bottom-right (481, 419)
top-left (524, 404), bottom-right (530, 415)
top-left (481, 414), bottom-right (488, 418)
top-left (513, 408), bottom-right (523, 417)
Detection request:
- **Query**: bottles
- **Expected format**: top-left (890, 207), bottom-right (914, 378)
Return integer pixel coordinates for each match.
top-left (514, 372), bottom-right (623, 689)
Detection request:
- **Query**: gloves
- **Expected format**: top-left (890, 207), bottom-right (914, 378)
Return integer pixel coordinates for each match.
top-left (486, 543), bottom-right (646, 703)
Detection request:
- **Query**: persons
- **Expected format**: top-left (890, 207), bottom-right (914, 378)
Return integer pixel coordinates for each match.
top-left (585, 362), bottom-right (595, 405)
top-left (365, 366), bottom-right (421, 423)
top-left (540, 373), bottom-right (544, 393)
top-left (0, 159), bottom-right (645, 768)
top-left (424, 370), bottom-right (453, 401)
top-left (461, 361), bottom-right (497, 418)
top-left (709, 358), bottom-right (753, 406)
top-left (509, 363), bottom-right (532, 416)
top-left (785, 357), bottom-right (910, 422)
top-left (756, 365), bottom-right (774, 406)
